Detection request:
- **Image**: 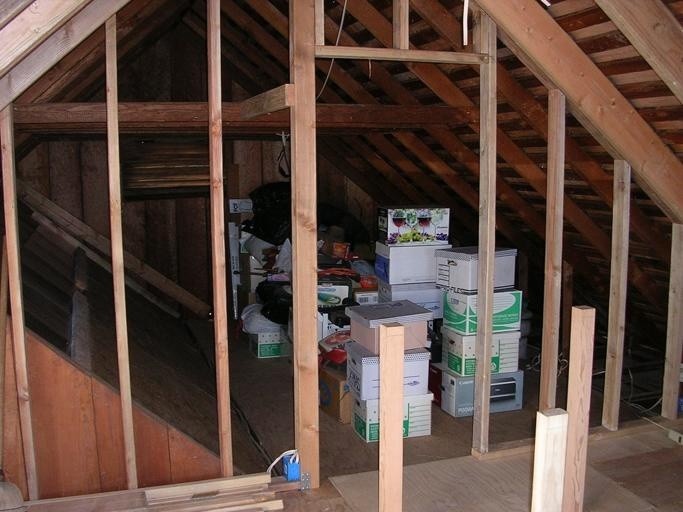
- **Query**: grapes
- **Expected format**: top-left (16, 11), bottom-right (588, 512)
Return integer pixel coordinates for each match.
top-left (387, 230), bottom-right (448, 245)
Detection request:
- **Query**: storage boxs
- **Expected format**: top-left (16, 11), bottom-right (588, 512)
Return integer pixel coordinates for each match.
top-left (319, 229), bottom-right (378, 424)
top-left (374, 205), bottom-right (453, 317)
top-left (345, 299), bottom-right (434, 443)
top-left (237, 249), bottom-right (292, 360)
top-left (429, 246), bottom-right (524, 418)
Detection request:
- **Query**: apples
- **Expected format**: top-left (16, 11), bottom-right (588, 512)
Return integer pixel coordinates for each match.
top-left (407, 213), bottom-right (416, 224)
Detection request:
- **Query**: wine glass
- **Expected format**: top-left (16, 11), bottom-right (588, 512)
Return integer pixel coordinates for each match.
top-left (392, 212), bottom-right (432, 245)
